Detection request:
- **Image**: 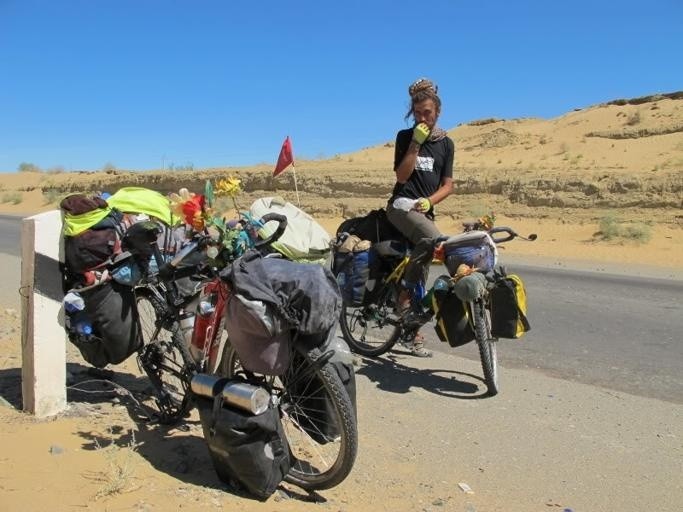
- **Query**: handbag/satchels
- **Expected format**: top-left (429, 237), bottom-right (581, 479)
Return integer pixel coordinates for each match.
top-left (332, 250), bottom-right (424, 308)
top-left (335, 207), bottom-right (406, 244)
top-left (283, 334), bottom-right (357, 444)
top-left (190, 370), bottom-right (296, 503)
top-left (487, 274), bottom-right (530, 340)
top-left (430, 286), bottom-right (476, 349)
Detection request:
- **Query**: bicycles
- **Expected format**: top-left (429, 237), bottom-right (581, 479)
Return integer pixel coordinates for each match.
top-left (332, 211), bottom-right (537, 397)
top-left (125, 210), bottom-right (359, 493)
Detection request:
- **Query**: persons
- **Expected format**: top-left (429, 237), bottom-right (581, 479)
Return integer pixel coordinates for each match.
top-left (381, 76), bottom-right (457, 359)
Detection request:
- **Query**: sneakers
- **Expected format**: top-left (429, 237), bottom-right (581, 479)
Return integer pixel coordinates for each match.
top-left (403, 336), bottom-right (432, 358)
top-left (392, 302), bottom-right (420, 329)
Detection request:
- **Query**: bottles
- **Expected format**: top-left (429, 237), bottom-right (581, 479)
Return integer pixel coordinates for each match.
top-left (190, 373), bottom-right (270, 415)
top-left (189, 301), bottom-right (219, 364)
top-left (419, 274), bottom-right (453, 307)
